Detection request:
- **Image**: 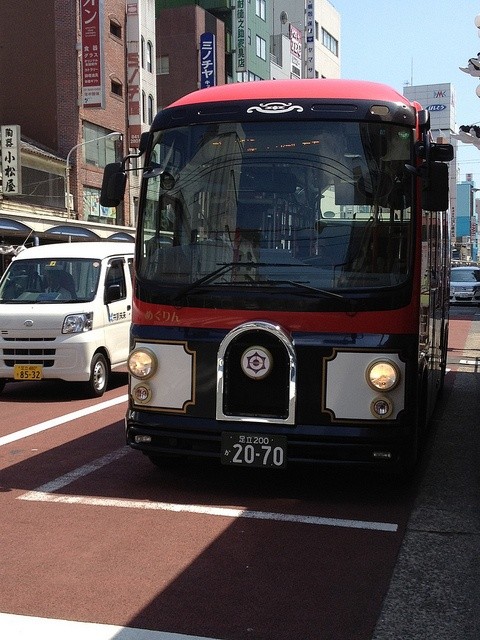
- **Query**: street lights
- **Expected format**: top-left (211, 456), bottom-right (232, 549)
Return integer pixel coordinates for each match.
top-left (60, 131), bottom-right (124, 243)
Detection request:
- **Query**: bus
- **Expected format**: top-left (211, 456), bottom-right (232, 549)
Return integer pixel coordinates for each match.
top-left (98, 77), bottom-right (455, 504)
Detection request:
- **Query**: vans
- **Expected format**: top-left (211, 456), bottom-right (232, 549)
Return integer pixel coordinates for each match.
top-left (2, 241), bottom-right (148, 401)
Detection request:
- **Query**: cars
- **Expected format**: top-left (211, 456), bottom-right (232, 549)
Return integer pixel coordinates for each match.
top-left (444, 265), bottom-right (480, 305)
